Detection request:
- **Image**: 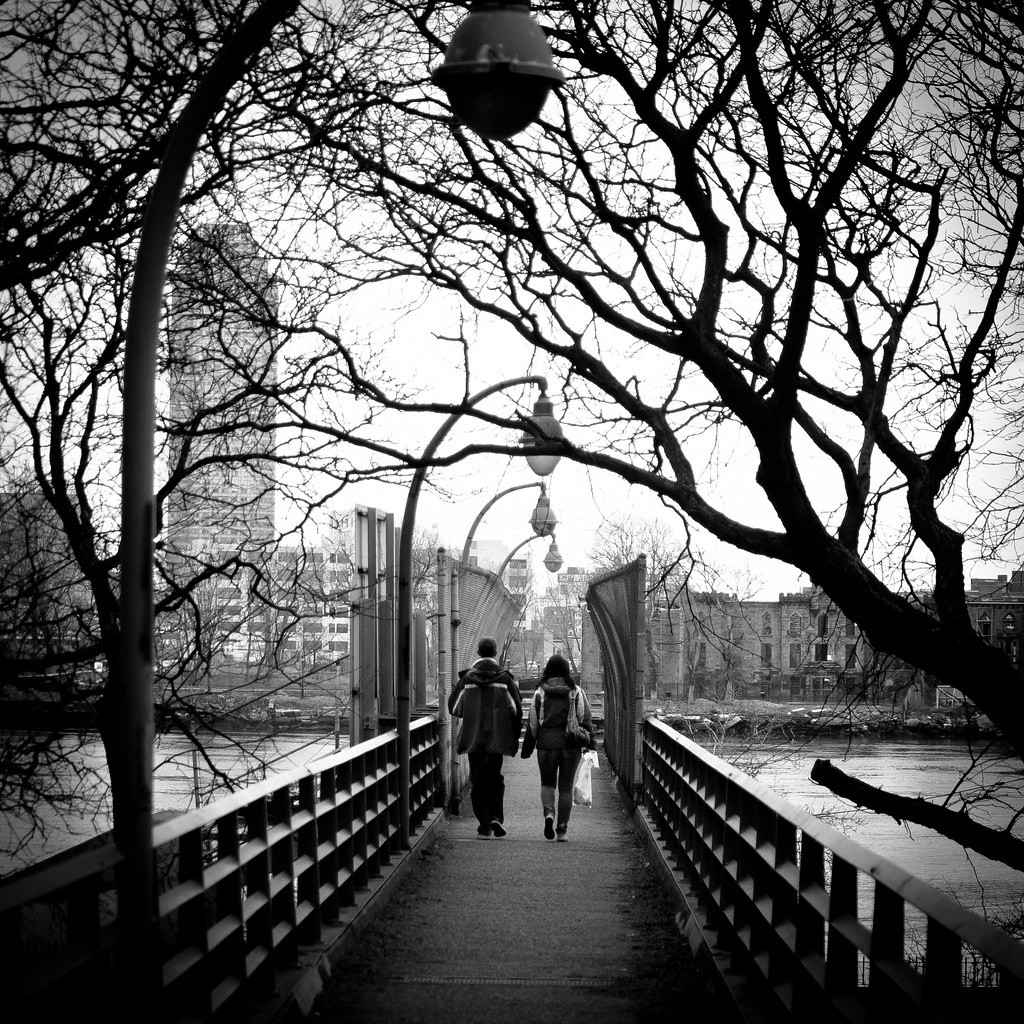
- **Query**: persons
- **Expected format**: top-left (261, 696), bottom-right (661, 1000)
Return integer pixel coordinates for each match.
top-left (521, 655), bottom-right (597, 841)
top-left (448, 638), bottom-right (523, 837)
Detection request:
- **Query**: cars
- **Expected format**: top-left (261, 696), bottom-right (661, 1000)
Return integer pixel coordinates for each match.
top-left (526, 660), bottom-right (538, 671)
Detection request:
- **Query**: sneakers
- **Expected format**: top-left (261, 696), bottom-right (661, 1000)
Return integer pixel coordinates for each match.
top-left (476, 819), bottom-right (507, 840)
top-left (543, 814), bottom-right (568, 841)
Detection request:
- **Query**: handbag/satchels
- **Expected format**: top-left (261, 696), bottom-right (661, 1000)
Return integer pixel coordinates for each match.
top-left (565, 685), bottom-right (591, 747)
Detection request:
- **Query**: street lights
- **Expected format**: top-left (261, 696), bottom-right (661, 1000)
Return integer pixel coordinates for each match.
top-left (450, 480), bottom-right (564, 817)
top-left (108, 0), bottom-right (563, 1023)
top-left (391, 376), bottom-right (571, 848)
